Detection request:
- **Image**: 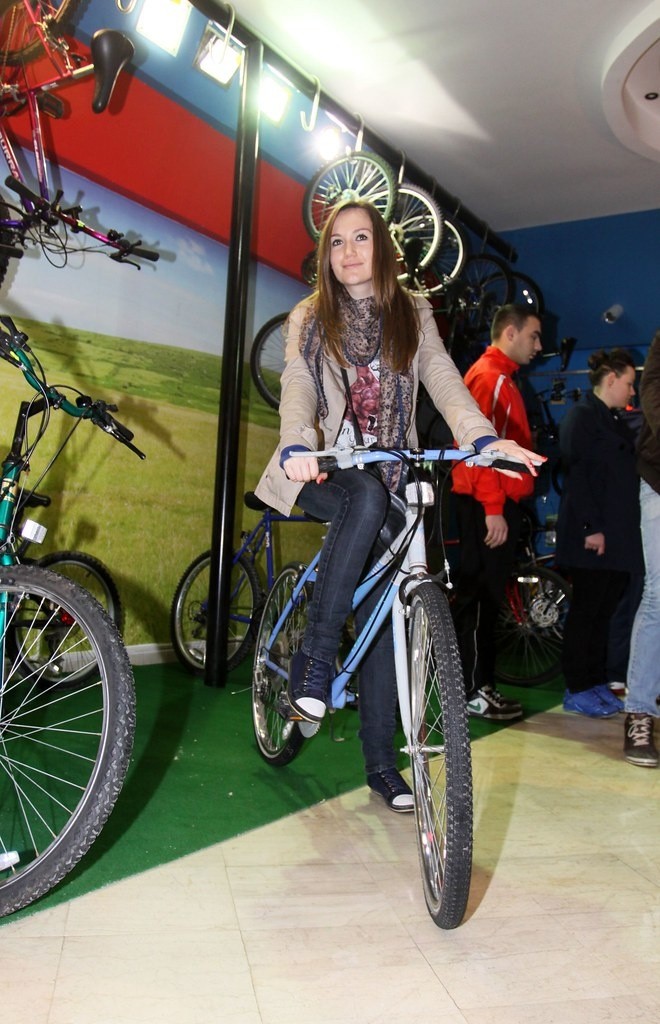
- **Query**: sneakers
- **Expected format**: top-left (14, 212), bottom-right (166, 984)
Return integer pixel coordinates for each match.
top-left (623, 714), bottom-right (659, 766)
top-left (593, 683), bottom-right (625, 713)
top-left (564, 686), bottom-right (619, 719)
top-left (465, 682), bottom-right (522, 720)
top-left (287, 649), bottom-right (330, 724)
top-left (367, 766), bottom-right (414, 812)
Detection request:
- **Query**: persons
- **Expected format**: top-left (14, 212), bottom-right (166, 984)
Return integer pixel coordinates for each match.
top-left (253, 199), bottom-right (548, 812)
top-left (450, 305), bottom-right (541, 719)
top-left (558, 352), bottom-right (635, 717)
top-left (625, 332), bottom-right (660, 768)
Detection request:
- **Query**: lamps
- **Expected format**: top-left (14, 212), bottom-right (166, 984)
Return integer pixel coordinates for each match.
top-left (193, 26), bottom-right (242, 91)
top-left (134, 0), bottom-right (192, 57)
top-left (258, 72), bottom-right (294, 129)
top-left (603, 304), bottom-right (623, 324)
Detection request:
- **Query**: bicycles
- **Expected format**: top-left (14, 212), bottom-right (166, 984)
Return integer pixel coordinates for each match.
top-left (1, 315), bottom-right (149, 919)
top-left (170, 447), bottom-right (542, 929)
top-left (0, 0), bottom-right (159, 291)
top-left (249, 148), bottom-right (570, 690)
top-left (7, 485), bottom-right (122, 690)
